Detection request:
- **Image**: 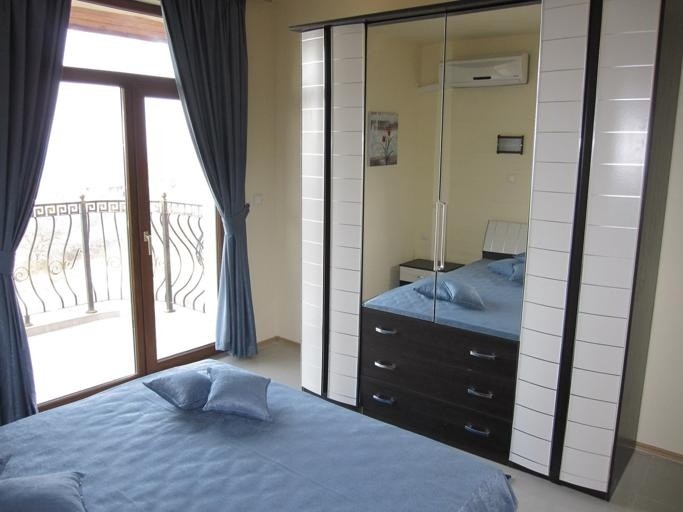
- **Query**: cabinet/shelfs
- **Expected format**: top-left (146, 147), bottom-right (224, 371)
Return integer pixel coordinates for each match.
top-left (356, 304), bottom-right (521, 467)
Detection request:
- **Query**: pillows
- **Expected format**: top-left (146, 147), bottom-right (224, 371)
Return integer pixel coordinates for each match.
top-left (487, 258), bottom-right (518, 276)
top-left (513, 252), bottom-right (525, 261)
top-left (411, 282), bottom-right (449, 301)
top-left (0, 469), bottom-right (89, 509)
top-left (440, 278), bottom-right (486, 310)
top-left (143, 372), bottom-right (214, 410)
top-left (203, 360), bottom-right (271, 418)
top-left (508, 263), bottom-right (525, 282)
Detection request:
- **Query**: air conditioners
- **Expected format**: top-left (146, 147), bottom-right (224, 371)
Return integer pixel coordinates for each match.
top-left (435, 53), bottom-right (533, 87)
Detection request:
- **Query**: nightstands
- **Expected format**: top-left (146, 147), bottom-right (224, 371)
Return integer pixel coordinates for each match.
top-left (396, 258), bottom-right (465, 287)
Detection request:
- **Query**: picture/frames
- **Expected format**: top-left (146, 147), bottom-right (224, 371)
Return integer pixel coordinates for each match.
top-left (493, 135), bottom-right (527, 156)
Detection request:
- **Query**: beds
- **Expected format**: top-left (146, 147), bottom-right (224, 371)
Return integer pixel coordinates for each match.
top-left (0, 350), bottom-right (521, 512)
top-left (360, 217), bottom-right (529, 342)
